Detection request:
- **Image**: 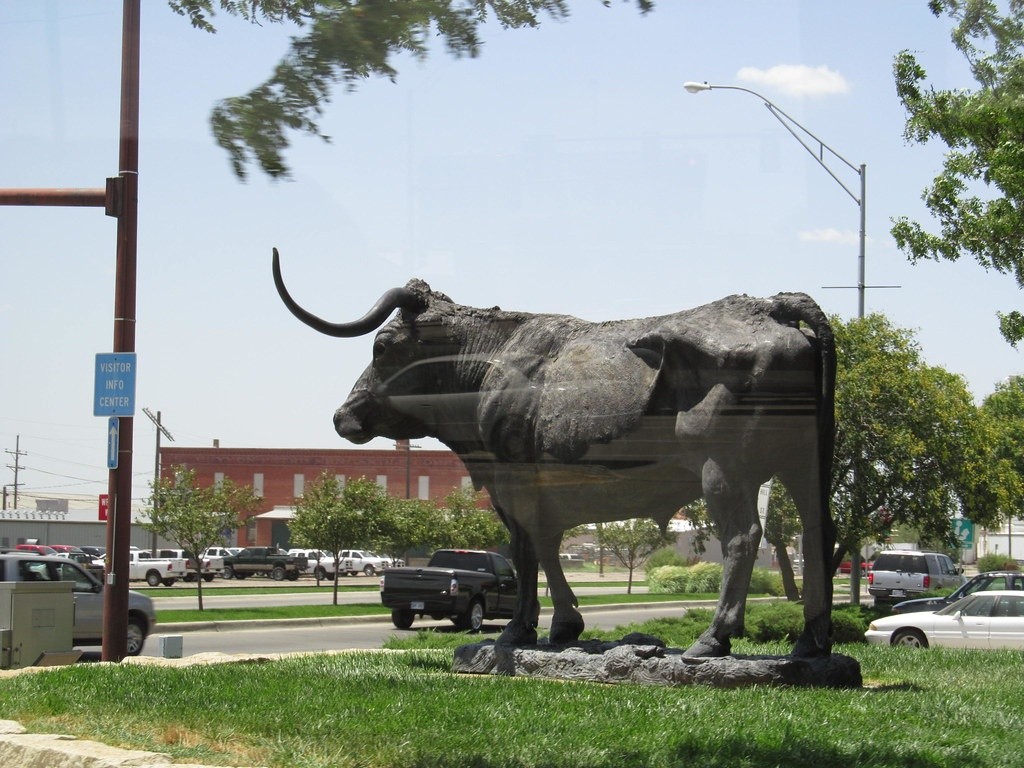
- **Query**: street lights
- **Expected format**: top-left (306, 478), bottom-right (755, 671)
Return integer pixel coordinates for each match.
top-left (683, 81), bottom-right (901, 609)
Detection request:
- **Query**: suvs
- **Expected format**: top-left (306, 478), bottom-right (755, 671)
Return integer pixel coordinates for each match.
top-left (867, 548), bottom-right (968, 605)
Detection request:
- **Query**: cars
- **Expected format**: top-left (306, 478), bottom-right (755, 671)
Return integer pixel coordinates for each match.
top-left (864, 590), bottom-right (1024, 654)
top-left (837, 550), bottom-right (874, 577)
top-left (559, 553), bottom-right (585, 562)
top-left (891, 570), bottom-right (1024, 615)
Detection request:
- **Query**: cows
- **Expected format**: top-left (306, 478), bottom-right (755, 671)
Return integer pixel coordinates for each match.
top-left (271, 245), bottom-right (837, 657)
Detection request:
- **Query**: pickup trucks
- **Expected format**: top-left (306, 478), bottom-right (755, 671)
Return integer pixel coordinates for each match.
top-left (380, 549), bottom-right (519, 633)
top-left (0, 545), bottom-right (405, 587)
top-left (1, 555), bottom-right (156, 655)
